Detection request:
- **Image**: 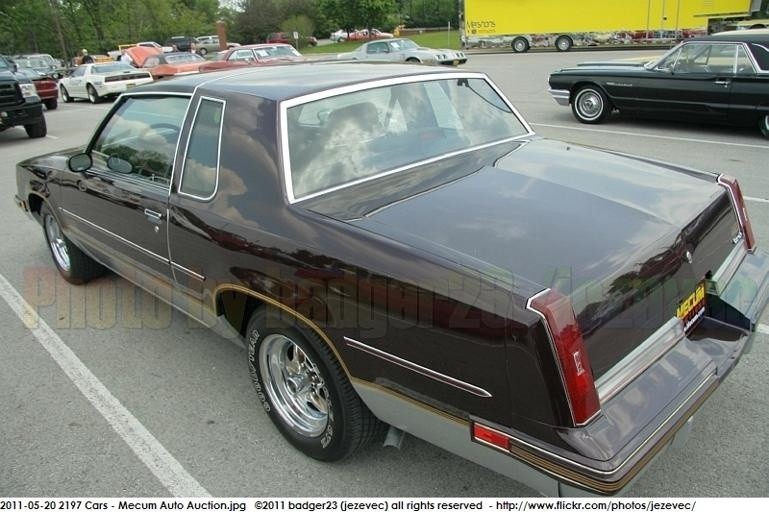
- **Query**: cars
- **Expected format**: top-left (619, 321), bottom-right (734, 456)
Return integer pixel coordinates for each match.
top-left (0, 27), bottom-right (468, 138)
top-left (17, 61), bottom-right (769, 497)
top-left (549, 33), bottom-right (769, 140)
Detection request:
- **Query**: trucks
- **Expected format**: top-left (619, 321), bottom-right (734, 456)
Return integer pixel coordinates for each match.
top-left (461, 1), bottom-right (769, 53)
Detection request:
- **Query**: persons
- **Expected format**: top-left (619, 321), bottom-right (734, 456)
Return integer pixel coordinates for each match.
top-left (81, 49), bottom-right (94, 63)
